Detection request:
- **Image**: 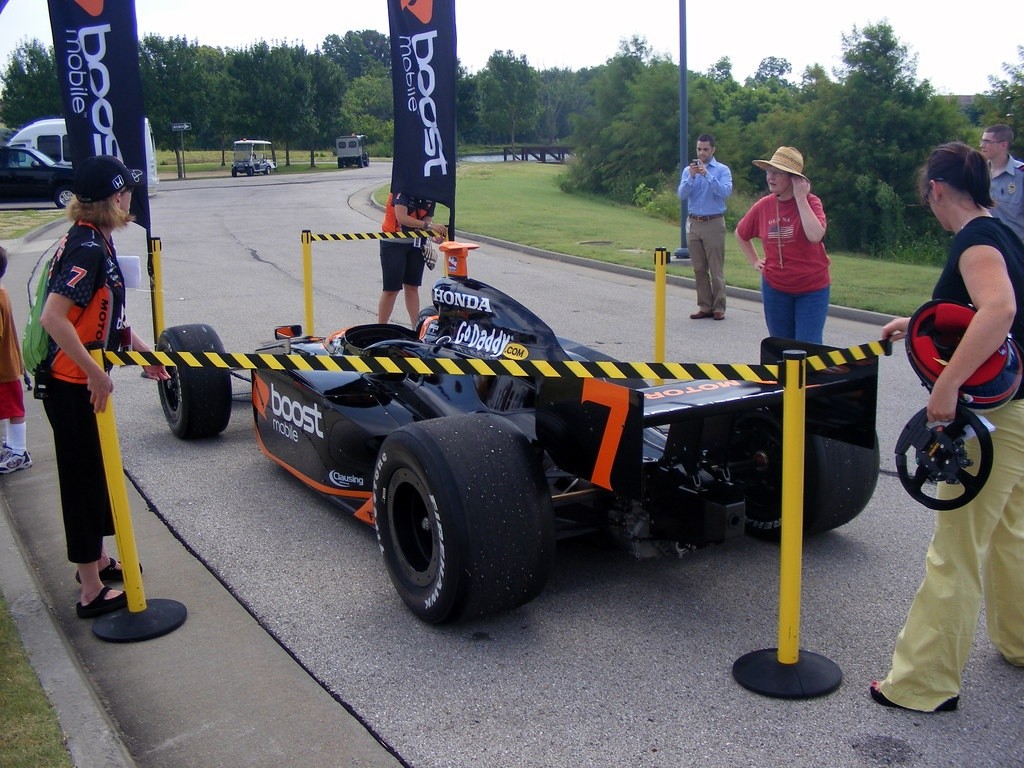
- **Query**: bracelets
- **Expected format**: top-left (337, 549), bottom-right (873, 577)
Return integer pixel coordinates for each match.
top-left (690, 174), bottom-right (696, 179)
top-left (423, 221), bottom-right (432, 231)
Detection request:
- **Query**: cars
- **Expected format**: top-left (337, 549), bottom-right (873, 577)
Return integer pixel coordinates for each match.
top-left (0, 146), bottom-right (78, 209)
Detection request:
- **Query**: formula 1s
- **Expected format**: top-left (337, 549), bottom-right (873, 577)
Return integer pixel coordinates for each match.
top-left (157, 239), bottom-right (882, 626)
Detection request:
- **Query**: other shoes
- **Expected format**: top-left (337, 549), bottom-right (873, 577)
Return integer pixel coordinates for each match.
top-left (870, 679), bottom-right (960, 713)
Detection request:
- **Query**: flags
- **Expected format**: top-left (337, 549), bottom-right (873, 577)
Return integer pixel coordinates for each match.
top-left (387, 0), bottom-right (457, 237)
top-left (47, 0), bottom-right (151, 230)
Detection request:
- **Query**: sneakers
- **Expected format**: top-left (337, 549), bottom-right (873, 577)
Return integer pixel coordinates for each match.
top-left (0, 442), bottom-right (33, 475)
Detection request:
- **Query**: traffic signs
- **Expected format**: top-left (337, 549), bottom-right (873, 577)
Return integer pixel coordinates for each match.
top-left (170, 124), bottom-right (191, 131)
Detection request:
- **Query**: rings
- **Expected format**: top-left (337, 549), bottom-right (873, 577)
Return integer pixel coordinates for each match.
top-left (941, 418), bottom-right (948, 422)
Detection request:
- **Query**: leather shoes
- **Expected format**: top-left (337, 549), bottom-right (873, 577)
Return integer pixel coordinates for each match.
top-left (690, 311), bottom-right (725, 322)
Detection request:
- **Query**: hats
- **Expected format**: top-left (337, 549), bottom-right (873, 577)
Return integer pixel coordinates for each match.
top-left (76, 155), bottom-right (146, 202)
top-left (752, 146), bottom-right (809, 182)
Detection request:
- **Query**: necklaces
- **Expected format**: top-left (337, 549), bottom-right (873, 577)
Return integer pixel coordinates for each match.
top-left (960, 215), bottom-right (993, 230)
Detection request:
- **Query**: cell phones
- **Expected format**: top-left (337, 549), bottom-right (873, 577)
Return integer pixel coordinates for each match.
top-left (693, 159), bottom-right (699, 167)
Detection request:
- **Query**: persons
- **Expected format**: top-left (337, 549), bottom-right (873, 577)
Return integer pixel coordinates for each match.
top-left (0, 246), bottom-right (32, 473)
top-left (870, 142), bottom-right (1024, 712)
top-left (378, 193), bottom-right (447, 332)
top-left (43, 155), bottom-right (171, 619)
top-left (735, 146), bottom-right (831, 344)
top-left (980, 124), bottom-right (1024, 245)
top-left (677, 134), bottom-right (732, 320)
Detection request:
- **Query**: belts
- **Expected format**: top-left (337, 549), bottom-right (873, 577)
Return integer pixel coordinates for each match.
top-left (689, 213), bottom-right (722, 221)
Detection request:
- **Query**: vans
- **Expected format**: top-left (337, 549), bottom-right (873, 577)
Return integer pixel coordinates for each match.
top-left (5, 118), bottom-right (159, 188)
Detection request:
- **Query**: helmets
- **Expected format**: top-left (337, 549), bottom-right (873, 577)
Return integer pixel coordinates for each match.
top-left (906, 300), bottom-right (1024, 411)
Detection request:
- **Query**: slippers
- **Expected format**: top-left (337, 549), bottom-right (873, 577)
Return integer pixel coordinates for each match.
top-left (75, 559), bottom-right (143, 619)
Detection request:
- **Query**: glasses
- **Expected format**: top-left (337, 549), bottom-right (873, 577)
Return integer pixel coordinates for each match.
top-left (120, 185), bottom-right (134, 196)
top-left (766, 169), bottom-right (786, 177)
top-left (924, 177), bottom-right (944, 201)
top-left (979, 138), bottom-right (1005, 145)
